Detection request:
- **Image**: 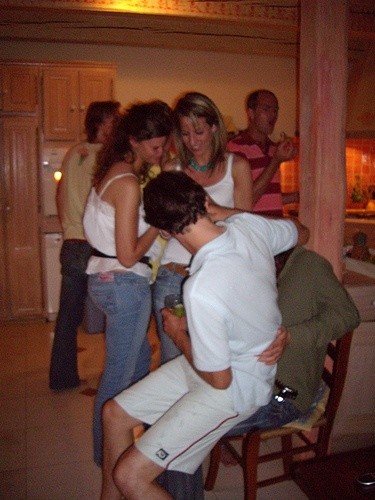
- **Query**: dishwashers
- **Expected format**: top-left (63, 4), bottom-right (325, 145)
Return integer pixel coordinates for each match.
top-left (44, 232), bottom-right (62, 322)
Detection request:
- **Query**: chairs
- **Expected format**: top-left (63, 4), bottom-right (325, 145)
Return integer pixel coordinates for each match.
top-left (202, 327), bottom-right (353, 500)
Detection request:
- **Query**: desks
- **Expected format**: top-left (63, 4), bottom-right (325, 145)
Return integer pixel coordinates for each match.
top-left (292, 446), bottom-right (375, 499)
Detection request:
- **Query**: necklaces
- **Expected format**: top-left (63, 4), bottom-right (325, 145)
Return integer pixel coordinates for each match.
top-left (187, 159), bottom-right (211, 170)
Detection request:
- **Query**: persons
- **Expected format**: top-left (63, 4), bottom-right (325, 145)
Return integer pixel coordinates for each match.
top-left (47, 89), bottom-right (361, 500)
top-left (99, 170), bottom-right (310, 500)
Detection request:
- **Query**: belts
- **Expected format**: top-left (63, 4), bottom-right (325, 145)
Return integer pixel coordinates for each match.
top-left (165, 261), bottom-right (188, 279)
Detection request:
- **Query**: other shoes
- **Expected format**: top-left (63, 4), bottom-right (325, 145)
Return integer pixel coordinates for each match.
top-left (50, 379), bottom-right (78, 390)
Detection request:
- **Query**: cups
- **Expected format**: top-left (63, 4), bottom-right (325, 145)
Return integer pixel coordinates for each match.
top-left (165, 294), bottom-right (186, 318)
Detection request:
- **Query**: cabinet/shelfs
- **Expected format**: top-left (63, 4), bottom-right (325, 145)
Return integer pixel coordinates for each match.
top-left (38, 57), bottom-right (119, 141)
top-left (0, 59), bottom-right (50, 325)
